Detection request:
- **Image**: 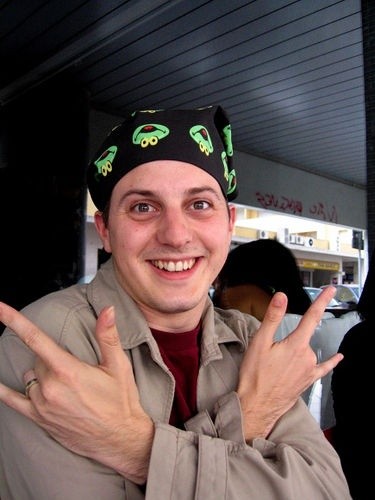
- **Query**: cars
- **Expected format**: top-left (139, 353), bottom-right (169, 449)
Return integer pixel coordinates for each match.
top-left (320, 284), bottom-right (364, 305)
top-left (303, 287), bottom-right (343, 309)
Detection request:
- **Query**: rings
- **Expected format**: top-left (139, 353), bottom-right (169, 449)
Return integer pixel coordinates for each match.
top-left (24, 378), bottom-right (39, 398)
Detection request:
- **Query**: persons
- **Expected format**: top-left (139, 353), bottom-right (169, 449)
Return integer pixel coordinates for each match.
top-left (0, 104), bottom-right (353, 500)
top-left (211, 237), bottom-right (375, 500)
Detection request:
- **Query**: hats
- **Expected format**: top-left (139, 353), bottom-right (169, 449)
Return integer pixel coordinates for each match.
top-left (216, 237), bottom-right (314, 316)
top-left (85, 106), bottom-right (238, 212)
